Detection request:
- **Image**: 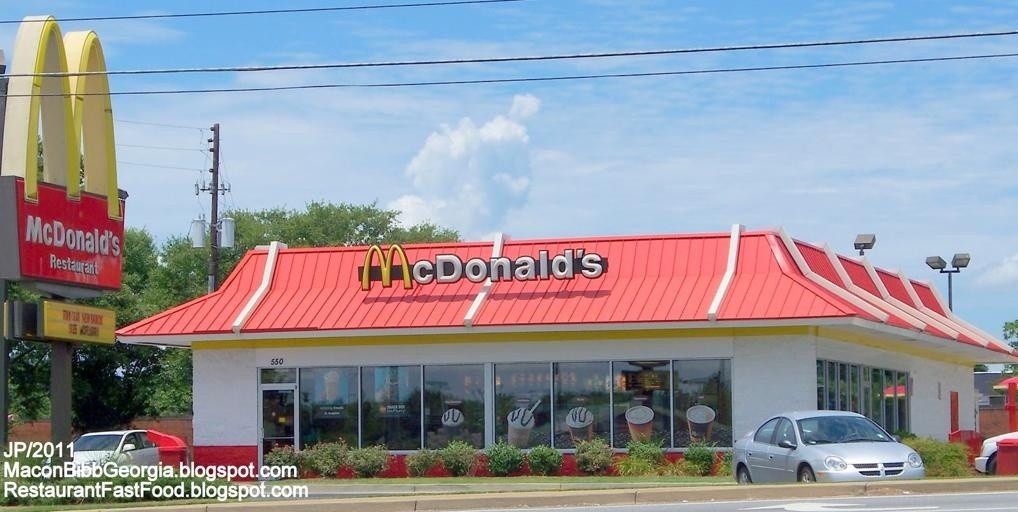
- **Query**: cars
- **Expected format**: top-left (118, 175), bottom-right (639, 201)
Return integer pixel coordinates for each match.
top-left (970, 429), bottom-right (1018, 477)
top-left (39, 428), bottom-right (161, 478)
top-left (731, 409), bottom-right (928, 484)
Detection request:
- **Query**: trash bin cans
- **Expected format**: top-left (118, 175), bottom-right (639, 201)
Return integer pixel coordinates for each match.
top-left (996, 438), bottom-right (1018, 476)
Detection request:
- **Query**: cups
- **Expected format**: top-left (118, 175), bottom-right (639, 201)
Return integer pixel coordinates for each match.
top-left (566, 416), bottom-right (594, 448)
top-left (439, 417), bottom-right (465, 447)
top-left (686, 406), bottom-right (715, 444)
top-left (624, 406), bottom-right (654, 443)
top-left (324, 373), bottom-right (337, 403)
top-left (507, 421), bottom-right (532, 449)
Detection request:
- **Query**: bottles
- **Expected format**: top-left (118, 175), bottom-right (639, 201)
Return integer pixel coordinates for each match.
top-left (382, 366), bottom-right (402, 405)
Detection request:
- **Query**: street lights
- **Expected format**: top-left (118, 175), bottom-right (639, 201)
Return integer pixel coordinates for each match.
top-left (924, 253), bottom-right (972, 313)
top-left (853, 232), bottom-right (878, 256)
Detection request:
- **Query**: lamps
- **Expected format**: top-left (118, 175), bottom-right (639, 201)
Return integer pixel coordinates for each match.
top-left (854, 234), bottom-right (876, 256)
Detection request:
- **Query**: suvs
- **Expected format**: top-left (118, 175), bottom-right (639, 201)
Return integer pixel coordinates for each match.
top-left (629, 385), bottom-right (682, 409)
